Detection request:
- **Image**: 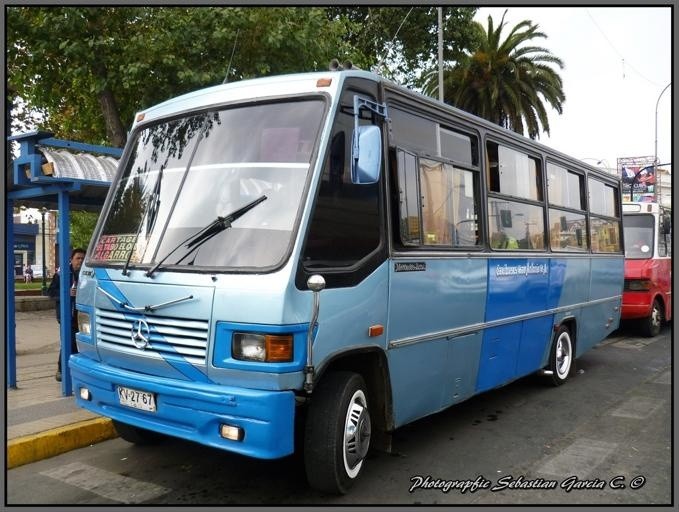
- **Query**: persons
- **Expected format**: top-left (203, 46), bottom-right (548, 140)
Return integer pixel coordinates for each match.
top-left (47, 249), bottom-right (86, 382)
top-left (638, 172), bottom-right (654, 192)
top-left (24, 265), bottom-right (33, 283)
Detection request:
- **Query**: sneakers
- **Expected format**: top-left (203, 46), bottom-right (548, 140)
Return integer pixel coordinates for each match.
top-left (56, 373), bottom-right (62, 381)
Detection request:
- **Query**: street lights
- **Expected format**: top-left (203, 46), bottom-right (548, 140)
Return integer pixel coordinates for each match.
top-left (38, 207), bottom-right (47, 297)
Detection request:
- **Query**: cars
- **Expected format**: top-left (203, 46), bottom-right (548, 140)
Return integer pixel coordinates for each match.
top-left (30, 264), bottom-right (47, 279)
